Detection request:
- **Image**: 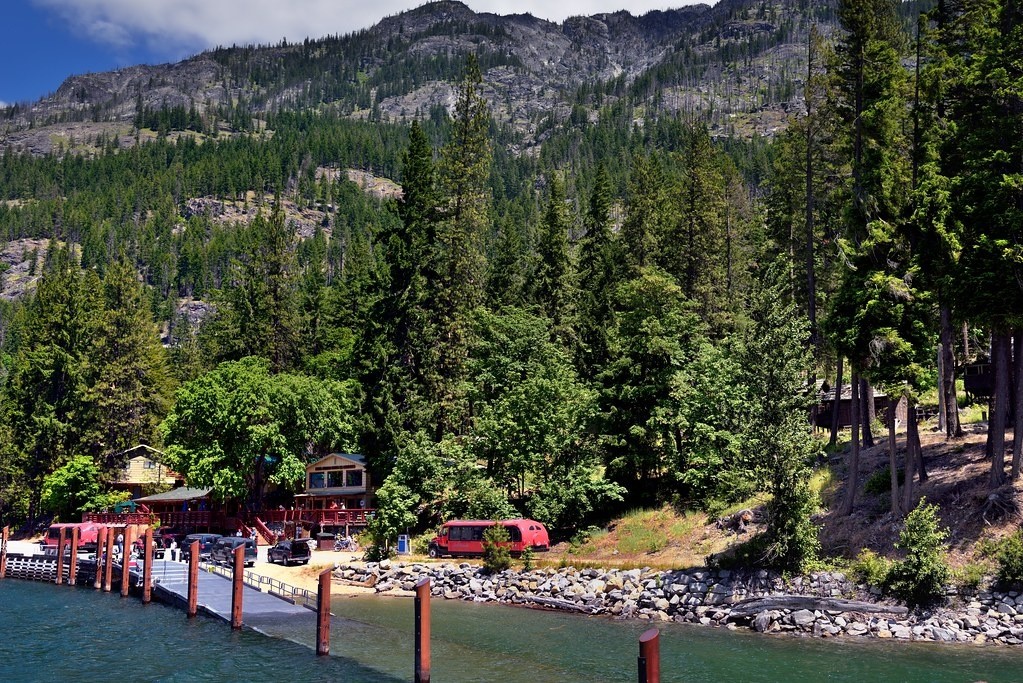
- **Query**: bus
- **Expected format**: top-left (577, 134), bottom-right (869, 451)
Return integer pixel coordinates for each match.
top-left (427, 519), bottom-right (550, 559)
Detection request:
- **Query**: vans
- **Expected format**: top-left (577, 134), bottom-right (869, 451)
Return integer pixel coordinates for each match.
top-left (40, 523), bottom-right (99, 551)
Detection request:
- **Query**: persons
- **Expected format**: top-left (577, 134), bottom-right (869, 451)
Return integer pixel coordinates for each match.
top-left (338, 503), bottom-right (346, 513)
top-left (236, 529), bottom-right (242, 537)
top-left (298, 504), bottom-right (305, 510)
top-left (330, 500), bottom-right (338, 509)
top-left (250, 527), bottom-right (256, 541)
top-left (117, 531), bottom-right (123, 554)
top-left (279, 505), bottom-right (285, 511)
top-left (170, 538), bottom-right (177, 561)
top-left (152, 538), bottom-right (157, 567)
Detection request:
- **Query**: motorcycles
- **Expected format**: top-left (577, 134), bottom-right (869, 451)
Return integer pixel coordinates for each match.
top-left (333, 535), bottom-right (357, 551)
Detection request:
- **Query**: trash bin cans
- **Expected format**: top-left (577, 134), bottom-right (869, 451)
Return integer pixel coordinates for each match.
top-left (397, 535), bottom-right (409, 553)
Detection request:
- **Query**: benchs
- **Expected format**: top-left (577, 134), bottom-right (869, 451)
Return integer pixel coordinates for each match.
top-left (357, 510), bottom-right (376, 521)
top-left (321, 513), bottom-right (352, 523)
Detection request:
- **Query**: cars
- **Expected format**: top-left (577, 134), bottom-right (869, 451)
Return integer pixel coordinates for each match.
top-left (180, 532), bottom-right (223, 557)
top-left (210, 536), bottom-right (259, 569)
top-left (266, 538), bottom-right (313, 565)
